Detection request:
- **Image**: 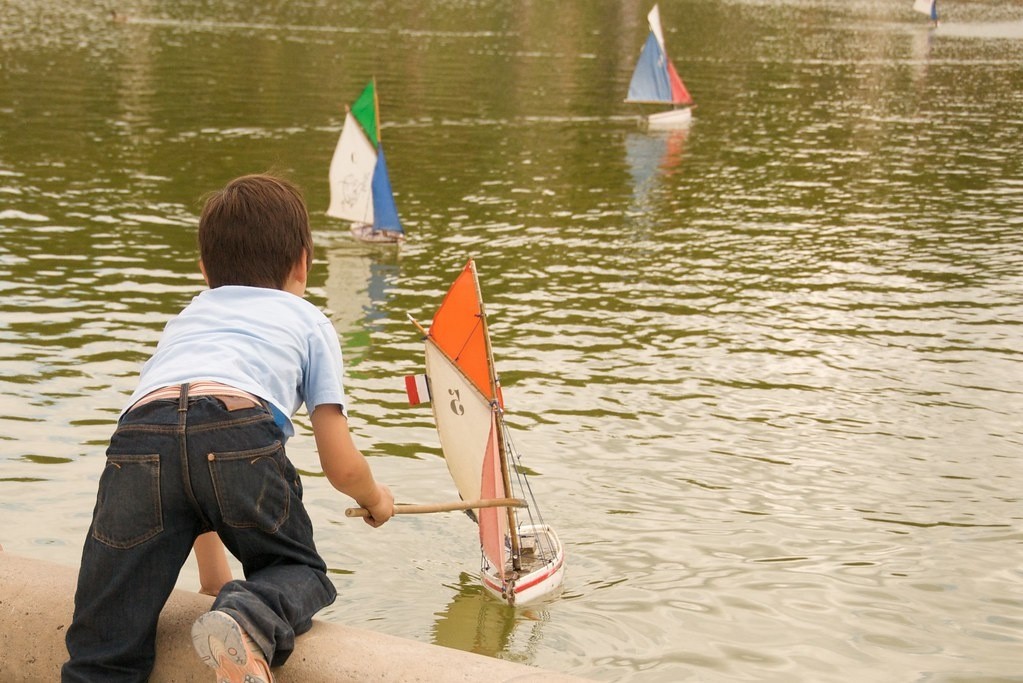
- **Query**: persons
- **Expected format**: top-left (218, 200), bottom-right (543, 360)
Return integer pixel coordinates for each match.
top-left (61, 173), bottom-right (395, 683)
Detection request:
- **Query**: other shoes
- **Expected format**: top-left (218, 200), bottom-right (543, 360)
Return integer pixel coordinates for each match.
top-left (191, 610), bottom-right (276, 683)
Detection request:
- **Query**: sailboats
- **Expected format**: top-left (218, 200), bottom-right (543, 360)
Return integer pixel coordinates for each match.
top-left (323, 73), bottom-right (407, 245)
top-left (913, 0), bottom-right (941, 32)
top-left (623, 3), bottom-right (698, 127)
top-left (405, 257), bottom-right (566, 605)
